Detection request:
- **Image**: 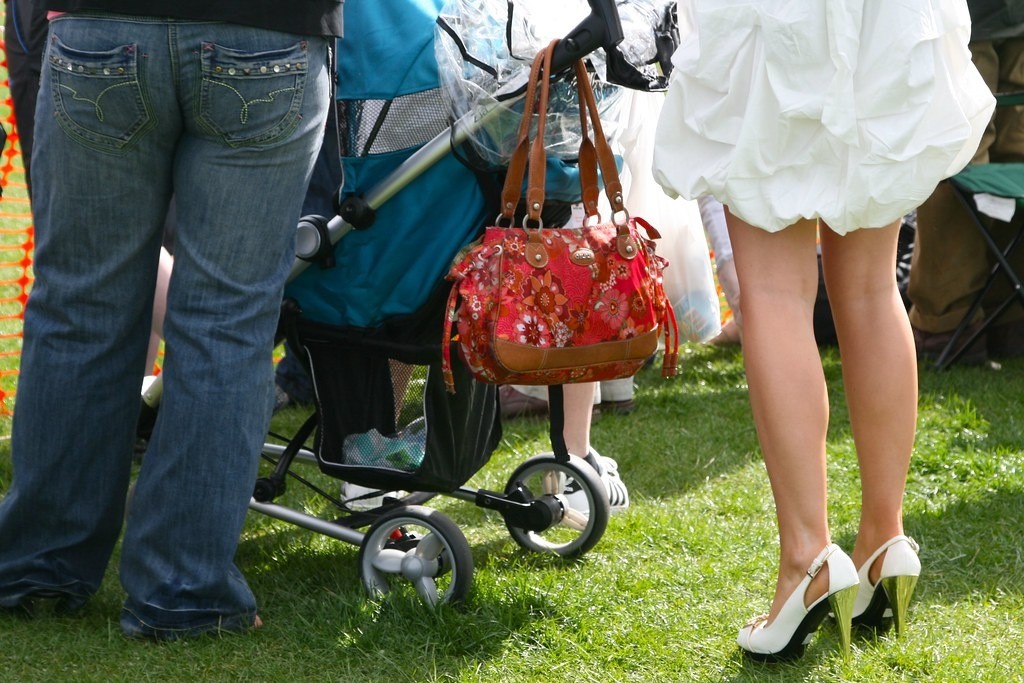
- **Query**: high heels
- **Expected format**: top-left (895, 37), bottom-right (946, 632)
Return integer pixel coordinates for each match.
top-left (828, 535), bottom-right (922, 639)
top-left (735, 543), bottom-right (861, 664)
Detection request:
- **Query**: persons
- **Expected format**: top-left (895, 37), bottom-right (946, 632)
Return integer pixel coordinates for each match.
top-left (0, 0), bottom-right (348, 638)
top-left (4, 0), bottom-right (916, 517)
top-left (907, 1), bottom-right (1024, 369)
top-left (649, 1), bottom-right (997, 668)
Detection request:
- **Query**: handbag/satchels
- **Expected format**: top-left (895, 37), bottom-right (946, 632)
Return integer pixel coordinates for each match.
top-left (440, 38), bottom-right (680, 393)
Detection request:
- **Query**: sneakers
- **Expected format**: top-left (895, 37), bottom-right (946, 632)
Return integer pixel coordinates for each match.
top-left (338, 481), bottom-right (408, 511)
top-left (558, 446), bottom-right (630, 517)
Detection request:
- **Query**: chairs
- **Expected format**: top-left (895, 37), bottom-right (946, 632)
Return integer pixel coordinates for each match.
top-left (931, 90), bottom-right (1024, 377)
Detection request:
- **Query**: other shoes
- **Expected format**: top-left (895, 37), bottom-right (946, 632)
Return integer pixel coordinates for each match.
top-left (497, 384), bottom-right (601, 416)
top-left (591, 399), bottom-right (636, 415)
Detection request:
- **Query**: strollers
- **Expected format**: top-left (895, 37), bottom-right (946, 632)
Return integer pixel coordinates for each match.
top-left (130, 1), bottom-right (679, 623)
